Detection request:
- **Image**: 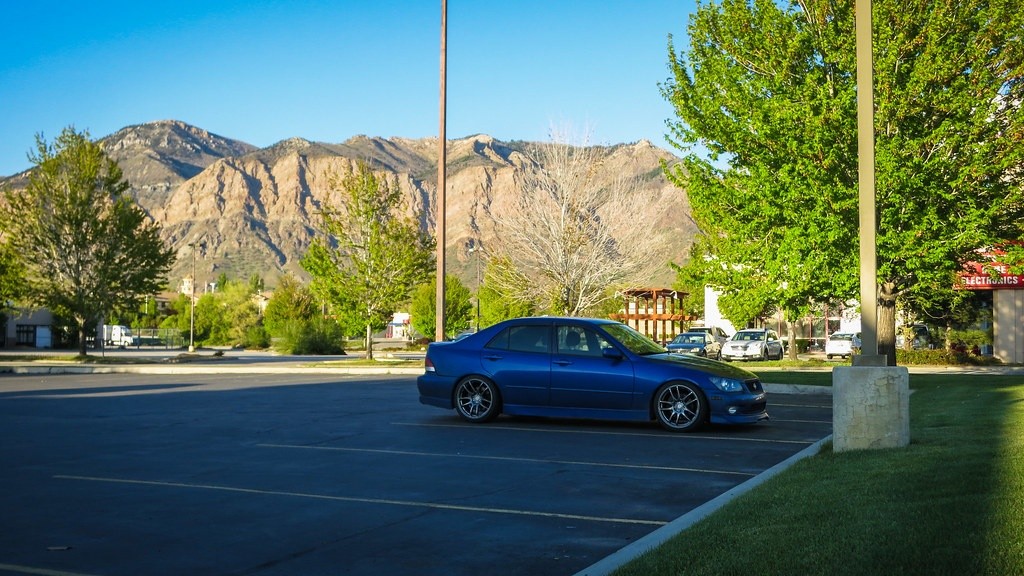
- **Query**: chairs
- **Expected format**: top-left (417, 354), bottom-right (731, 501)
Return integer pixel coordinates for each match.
top-left (565, 332), bottom-right (585, 355)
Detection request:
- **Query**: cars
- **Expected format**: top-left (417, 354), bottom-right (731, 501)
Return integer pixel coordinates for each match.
top-left (415, 316), bottom-right (769, 433)
top-left (721, 328), bottom-right (784, 363)
top-left (664, 331), bottom-right (720, 361)
top-left (824, 328), bottom-right (863, 358)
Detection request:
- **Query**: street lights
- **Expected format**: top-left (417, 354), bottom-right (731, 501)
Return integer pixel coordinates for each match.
top-left (188, 243), bottom-right (196, 351)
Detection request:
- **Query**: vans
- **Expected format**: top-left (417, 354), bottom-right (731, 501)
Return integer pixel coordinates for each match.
top-left (689, 326), bottom-right (731, 348)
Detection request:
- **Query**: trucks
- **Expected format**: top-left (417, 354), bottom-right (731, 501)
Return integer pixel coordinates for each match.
top-left (102, 324), bottom-right (133, 347)
